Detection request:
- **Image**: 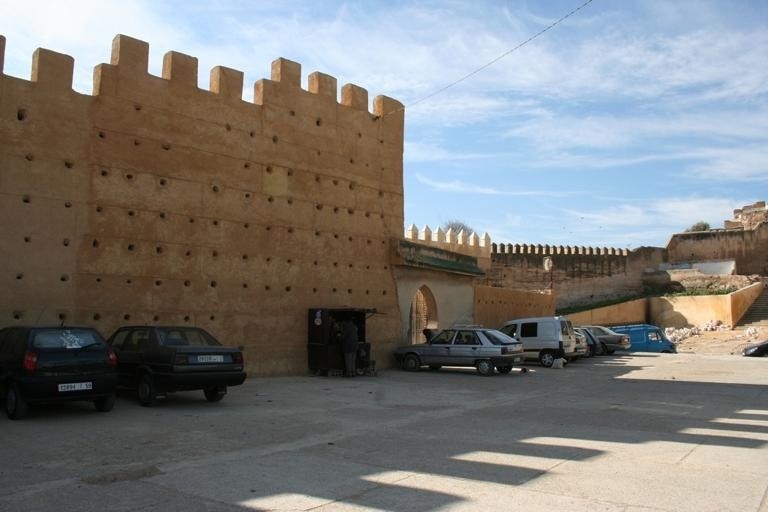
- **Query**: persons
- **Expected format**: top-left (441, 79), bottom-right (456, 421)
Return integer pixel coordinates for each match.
top-left (337, 316), bottom-right (361, 378)
top-left (422, 327), bottom-right (435, 342)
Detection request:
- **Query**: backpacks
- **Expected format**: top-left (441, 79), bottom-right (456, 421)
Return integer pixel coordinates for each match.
top-left (341, 327), bottom-right (357, 353)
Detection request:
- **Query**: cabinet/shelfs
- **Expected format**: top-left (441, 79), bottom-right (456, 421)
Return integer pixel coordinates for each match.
top-left (308, 308), bottom-right (378, 377)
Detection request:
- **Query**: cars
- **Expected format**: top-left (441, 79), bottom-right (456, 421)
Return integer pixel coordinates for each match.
top-left (742, 340), bottom-right (768, 357)
top-left (396, 326), bottom-right (632, 376)
top-left (0, 325), bottom-right (247, 419)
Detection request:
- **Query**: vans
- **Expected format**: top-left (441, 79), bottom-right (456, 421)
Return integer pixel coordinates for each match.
top-left (609, 324), bottom-right (677, 353)
top-left (499, 316), bottom-right (576, 368)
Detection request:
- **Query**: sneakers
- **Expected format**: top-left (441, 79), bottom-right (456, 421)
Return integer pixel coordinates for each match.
top-left (345, 370), bottom-right (357, 377)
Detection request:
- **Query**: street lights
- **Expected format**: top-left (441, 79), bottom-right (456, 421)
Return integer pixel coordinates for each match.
top-left (544, 256), bottom-right (553, 289)
